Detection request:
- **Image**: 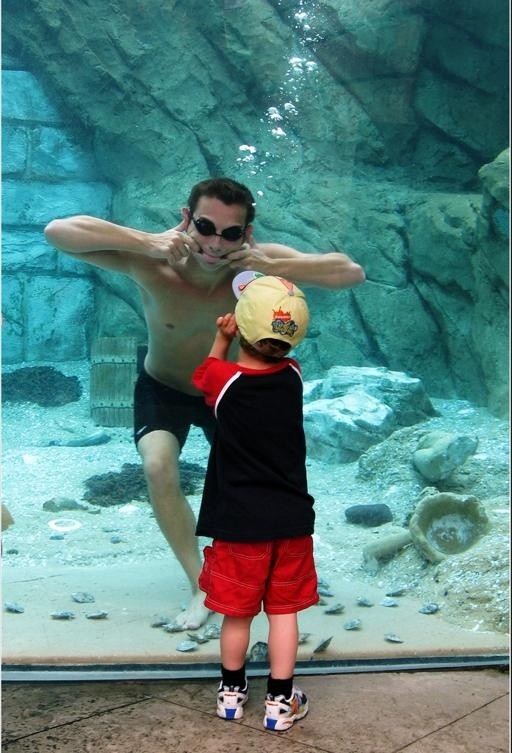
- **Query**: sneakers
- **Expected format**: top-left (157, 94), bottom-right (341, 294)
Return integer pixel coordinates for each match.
top-left (216, 676), bottom-right (249, 719)
top-left (262, 687), bottom-right (309, 731)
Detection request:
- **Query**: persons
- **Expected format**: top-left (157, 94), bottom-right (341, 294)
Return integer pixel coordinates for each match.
top-left (44, 175), bottom-right (365, 633)
top-left (190, 270), bottom-right (323, 731)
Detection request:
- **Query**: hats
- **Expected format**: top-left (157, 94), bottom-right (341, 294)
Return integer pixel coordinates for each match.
top-left (233, 271), bottom-right (309, 355)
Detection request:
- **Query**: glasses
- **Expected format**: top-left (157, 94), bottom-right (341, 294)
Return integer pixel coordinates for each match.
top-left (190, 216), bottom-right (245, 241)
